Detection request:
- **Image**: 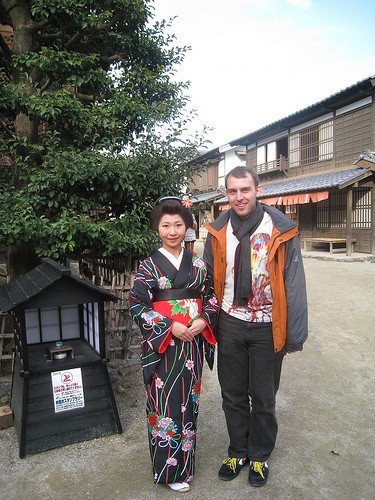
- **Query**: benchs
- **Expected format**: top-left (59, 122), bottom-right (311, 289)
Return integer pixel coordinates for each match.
top-left (303, 238), bottom-right (357, 254)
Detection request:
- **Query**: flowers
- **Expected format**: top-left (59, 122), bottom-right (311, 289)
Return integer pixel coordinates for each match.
top-left (182, 198), bottom-right (194, 208)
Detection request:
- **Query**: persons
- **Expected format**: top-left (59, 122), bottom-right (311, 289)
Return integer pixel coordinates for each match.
top-left (202, 166), bottom-right (309, 487)
top-left (183, 214), bottom-right (198, 251)
top-left (128, 197), bottom-right (218, 492)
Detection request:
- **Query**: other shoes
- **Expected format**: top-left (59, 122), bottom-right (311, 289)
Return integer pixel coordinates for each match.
top-left (167, 482), bottom-right (190, 493)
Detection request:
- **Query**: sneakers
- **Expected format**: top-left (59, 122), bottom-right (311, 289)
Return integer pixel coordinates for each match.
top-left (217, 457), bottom-right (248, 481)
top-left (249, 460), bottom-right (268, 486)
